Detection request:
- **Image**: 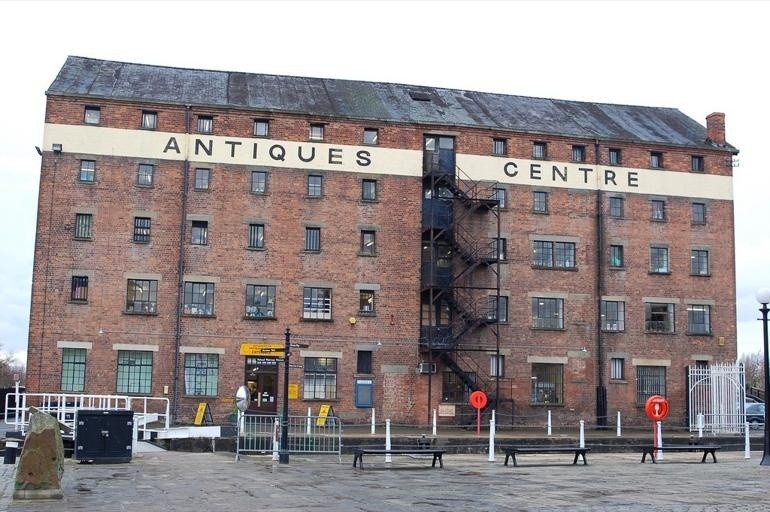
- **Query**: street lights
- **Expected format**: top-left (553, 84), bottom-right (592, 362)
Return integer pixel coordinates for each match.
top-left (756, 288), bottom-right (769, 463)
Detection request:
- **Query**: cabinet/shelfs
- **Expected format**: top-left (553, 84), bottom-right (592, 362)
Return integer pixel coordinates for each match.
top-left (74, 410), bottom-right (134, 464)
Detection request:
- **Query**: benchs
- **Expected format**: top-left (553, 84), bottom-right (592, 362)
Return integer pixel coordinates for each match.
top-left (638, 444), bottom-right (722, 463)
top-left (500, 445), bottom-right (591, 467)
top-left (352, 447), bottom-right (446, 470)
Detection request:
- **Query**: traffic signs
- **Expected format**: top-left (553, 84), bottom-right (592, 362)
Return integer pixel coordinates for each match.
top-left (239, 342), bottom-right (309, 368)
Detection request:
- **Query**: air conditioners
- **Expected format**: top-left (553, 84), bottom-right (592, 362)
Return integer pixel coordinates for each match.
top-left (419, 363), bottom-right (437, 375)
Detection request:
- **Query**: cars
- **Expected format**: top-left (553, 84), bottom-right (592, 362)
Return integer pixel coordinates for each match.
top-left (745, 402), bottom-right (765, 429)
